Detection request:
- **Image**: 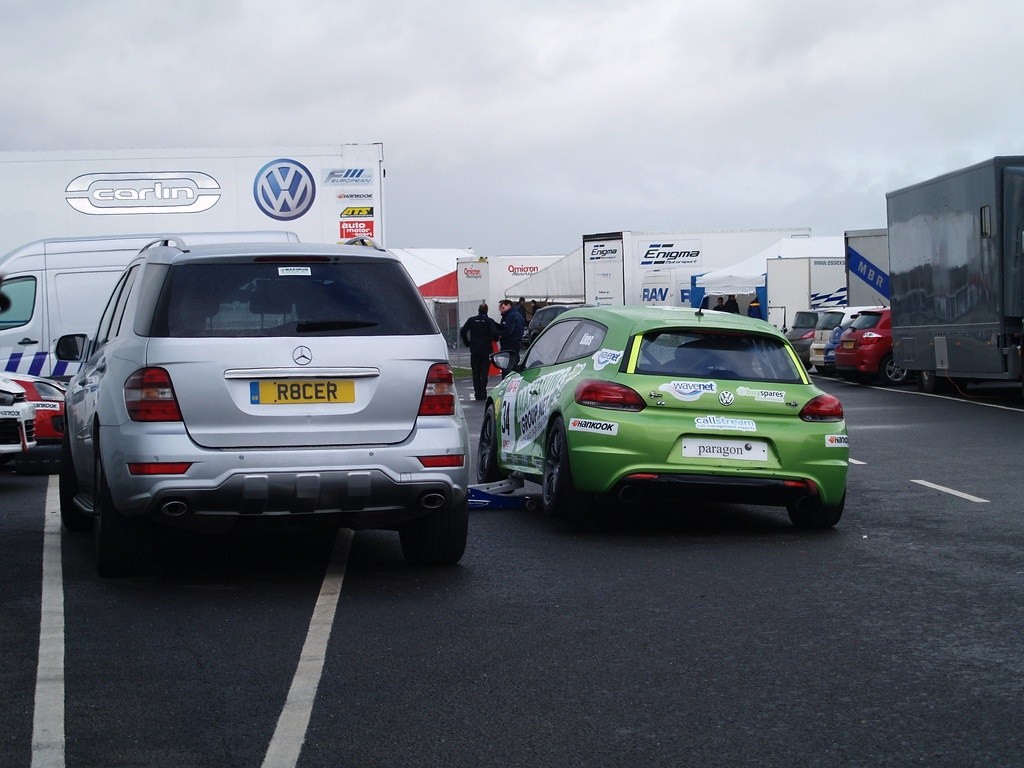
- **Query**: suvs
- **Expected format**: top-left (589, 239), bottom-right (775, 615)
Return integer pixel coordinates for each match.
top-left (54, 237), bottom-right (472, 578)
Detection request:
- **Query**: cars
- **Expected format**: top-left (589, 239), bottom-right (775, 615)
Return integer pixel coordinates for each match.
top-left (1, 369), bottom-right (67, 441)
top-left (808, 306), bottom-right (883, 376)
top-left (823, 314), bottom-right (863, 380)
top-left (786, 307), bottom-right (830, 372)
top-left (0, 374), bottom-right (38, 453)
top-left (477, 305), bottom-right (852, 532)
top-left (528, 305), bottom-right (596, 344)
top-left (833, 306), bottom-right (915, 386)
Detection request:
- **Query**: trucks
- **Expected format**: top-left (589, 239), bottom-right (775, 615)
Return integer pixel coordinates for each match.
top-left (841, 228), bottom-right (892, 308)
top-left (884, 154), bottom-right (1024, 396)
top-left (767, 255), bottom-right (847, 337)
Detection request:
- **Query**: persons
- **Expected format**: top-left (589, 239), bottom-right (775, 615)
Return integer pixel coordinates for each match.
top-left (721, 295), bottom-right (739, 314)
top-left (515, 297), bottom-right (526, 324)
top-left (523, 299), bottom-right (542, 338)
top-left (712, 296), bottom-right (724, 311)
top-left (747, 297), bottom-right (761, 319)
top-left (459, 304), bottom-right (502, 402)
top-left (489, 300), bottom-right (506, 352)
top-left (498, 300), bottom-right (525, 381)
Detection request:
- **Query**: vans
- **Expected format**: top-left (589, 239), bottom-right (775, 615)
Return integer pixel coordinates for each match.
top-left (1, 230), bottom-right (303, 385)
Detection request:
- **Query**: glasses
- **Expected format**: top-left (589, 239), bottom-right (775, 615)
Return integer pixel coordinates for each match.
top-left (501, 305), bottom-right (508, 309)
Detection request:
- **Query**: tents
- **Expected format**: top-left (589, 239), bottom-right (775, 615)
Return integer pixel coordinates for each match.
top-left (416, 269), bottom-right (459, 335)
top-left (505, 245), bottom-right (585, 304)
top-left (695, 236), bottom-right (847, 296)
